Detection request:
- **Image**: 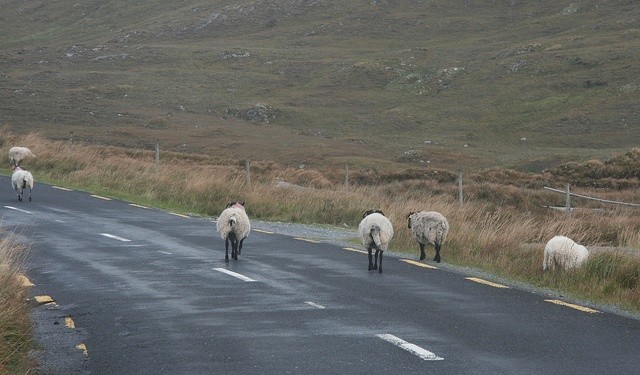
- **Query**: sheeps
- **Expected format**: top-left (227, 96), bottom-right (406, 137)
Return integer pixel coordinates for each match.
top-left (542, 234), bottom-right (590, 274)
top-left (359, 208), bottom-right (394, 274)
top-left (216, 201), bottom-right (251, 263)
top-left (9, 146), bottom-right (36, 166)
top-left (11, 161), bottom-right (34, 203)
top-left (406, 210), bottom-right (450, 264)
